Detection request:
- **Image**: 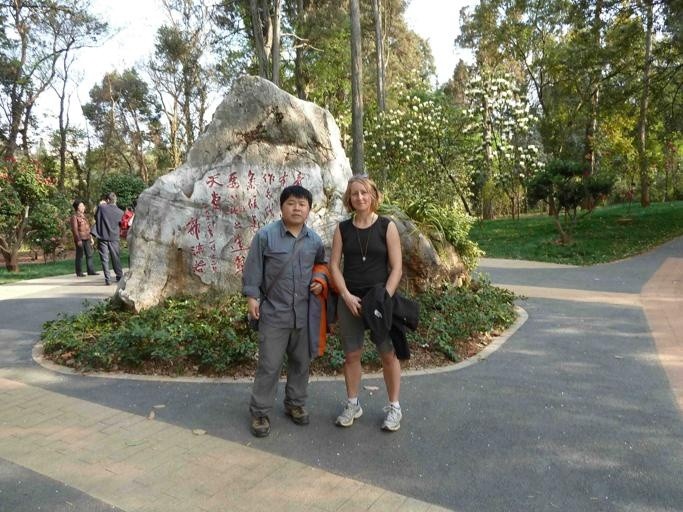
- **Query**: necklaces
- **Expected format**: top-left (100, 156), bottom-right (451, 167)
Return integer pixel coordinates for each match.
top-left (352, 210), bottom-right (374, 261)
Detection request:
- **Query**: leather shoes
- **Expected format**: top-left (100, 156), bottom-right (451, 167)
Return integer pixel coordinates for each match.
top-left (76, 270), bottom-right (101, 276)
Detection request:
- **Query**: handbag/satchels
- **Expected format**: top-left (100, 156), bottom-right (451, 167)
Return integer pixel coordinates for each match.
top-left (243, 308), bottom-right (260, 343)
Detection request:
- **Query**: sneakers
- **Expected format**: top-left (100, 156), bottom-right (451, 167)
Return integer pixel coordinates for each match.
top-left (283, 403), bottom-right (310, 426)
top-left (379, 402), bottom-right (403, 432)
top-left (250, 410), bottom-right (272, 437)
top-left (334, 399), bottom-right (364, 427)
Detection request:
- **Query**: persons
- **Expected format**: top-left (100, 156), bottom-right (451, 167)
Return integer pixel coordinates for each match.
top-left (70, 199), bottom-right (100, 277)
top-left (89, 191), bottom-right (125, 286)
top-left (242, 187), bottom-right (327, 438)
top-left (127, 215), bottom-right (133, 268)
top-left (328, 176), bottom-right (402, 434)
top-left (91, 199), bottom-right (106, 220)
